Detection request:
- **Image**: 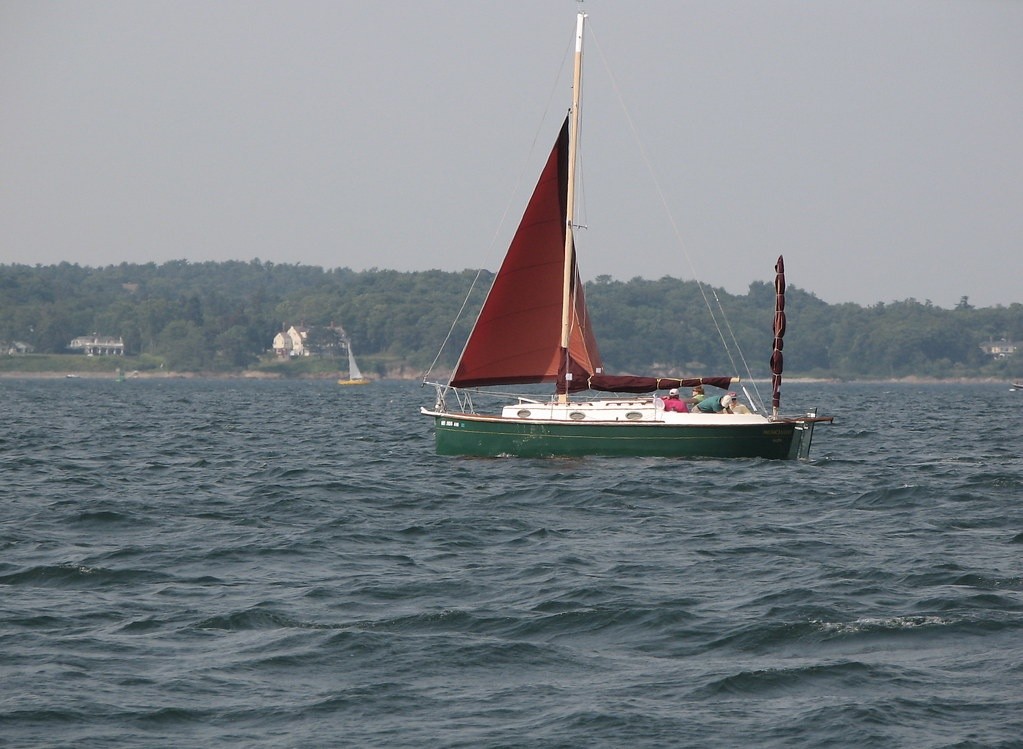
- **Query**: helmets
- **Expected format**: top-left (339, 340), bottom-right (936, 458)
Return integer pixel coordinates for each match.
top-left (669, 388), bottom-right (680, 396)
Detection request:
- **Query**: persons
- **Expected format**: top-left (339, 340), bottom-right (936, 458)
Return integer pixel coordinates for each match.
top-left (691, 394), bottom-right (734, 415)
top-left (678, 386), bottom-right (708, 406)
top-left (721, 392), bottom-right (752, 414)
top-left (663, 389), bottom-right (689, 413)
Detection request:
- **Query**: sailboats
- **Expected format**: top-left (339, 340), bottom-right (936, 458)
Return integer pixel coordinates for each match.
top-left (337, 342), bottom-right (371, 385)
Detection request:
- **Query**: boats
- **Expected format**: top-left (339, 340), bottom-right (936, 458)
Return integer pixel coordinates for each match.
top-left (1011, 381), bottom-right (1023, 389)
top-left (419, 12), bottom-right (839, 460)
top-left (66, 374), bottom-right (80, 378)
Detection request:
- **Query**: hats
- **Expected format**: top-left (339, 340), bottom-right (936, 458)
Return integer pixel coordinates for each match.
top-left (721, 395), bottom-right (732, 408)
top-left (728, 392), bottom-right (738, 399)
